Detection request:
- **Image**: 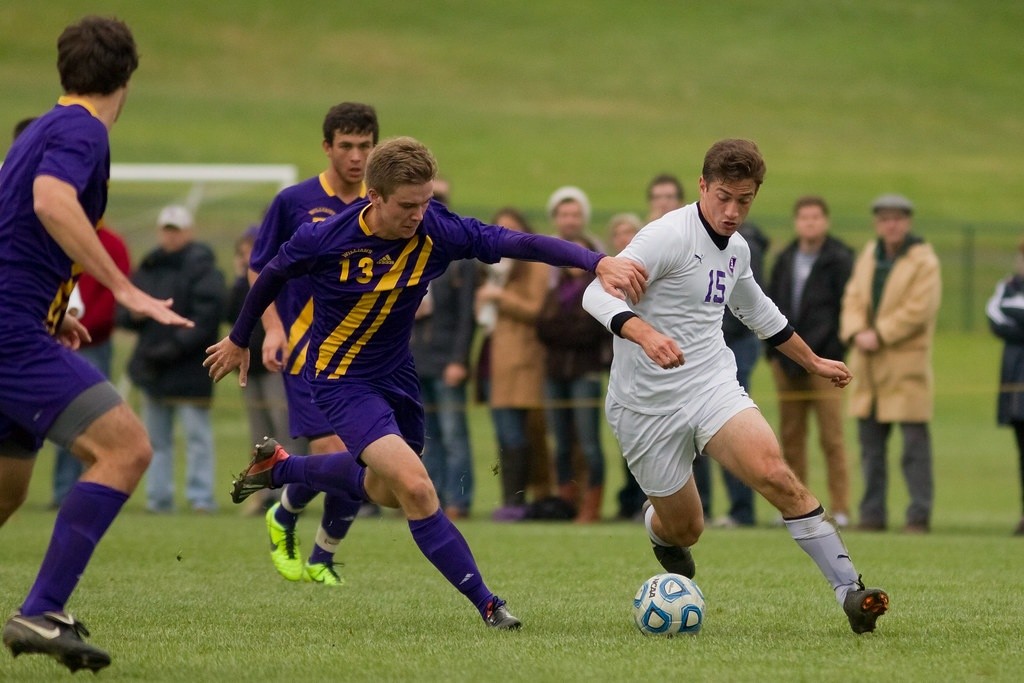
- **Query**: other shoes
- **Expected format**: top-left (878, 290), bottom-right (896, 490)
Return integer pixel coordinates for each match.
top-left (492, 507), bottom-right (526, 520)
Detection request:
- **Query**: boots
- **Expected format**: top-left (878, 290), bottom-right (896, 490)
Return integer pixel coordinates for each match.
top-left (557, 483), bottom-right (603, 523)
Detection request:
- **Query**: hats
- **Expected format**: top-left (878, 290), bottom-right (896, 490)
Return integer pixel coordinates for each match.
top-left (158, 204), bottom-right (195, 230)
top-left (872, 193), bottom-right (913, 215)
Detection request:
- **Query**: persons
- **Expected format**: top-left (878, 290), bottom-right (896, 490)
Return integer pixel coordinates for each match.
top-left (408, 178), bottom-right (490, 521)
top-left (985, 240), bottom-right (1024, 536)
top-left (647, 174), bottom-right (684, 221)
top-left (0, 14), bottom-right (196, 674)
top-left (693, 218), bottom-right (772, 528)
top-left (475, 205), bottom-right (551, 522)
top-left (224, 225), bottom-right (310, 518)
top-left (536, 238), bottom-right (608, 525)
top-left (119, 203), bottom-right (226, 513)
top-left (839, 193), bottom-right (943, 536)
top-left (765, 195), bottom-right (857, 529)
top-left (203, 136), bottom-right (649, 629)
top-left (610, 213), bottom-right (649, 528)
top-left (46, 217), bottom-right (129, 512)
top-left (545, 186), bottom-right (610, 285)
top-left (248, 101), bottom-right (380, 586)
top-left (581, 138), bottom-right (889, 635)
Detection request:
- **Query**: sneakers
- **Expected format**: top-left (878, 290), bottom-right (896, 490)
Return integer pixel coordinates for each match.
top-left (842, 574), bottom-right (889, 635)
top-left (302, 555), bottom-right (345, 586)
top-left (485, 596), bottom-right (521, 629)
top-left (642, 499), bottom-right (696, 579)
top-left (229, 436), bottom-right (289, 504)
top-left (265, 501), bottom-right (302, 580)
top-left (3, 609), bottom-right (111, 673)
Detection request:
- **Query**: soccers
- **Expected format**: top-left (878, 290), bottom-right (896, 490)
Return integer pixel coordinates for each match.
top-left (632, 571), bottom-right (707, 638)
